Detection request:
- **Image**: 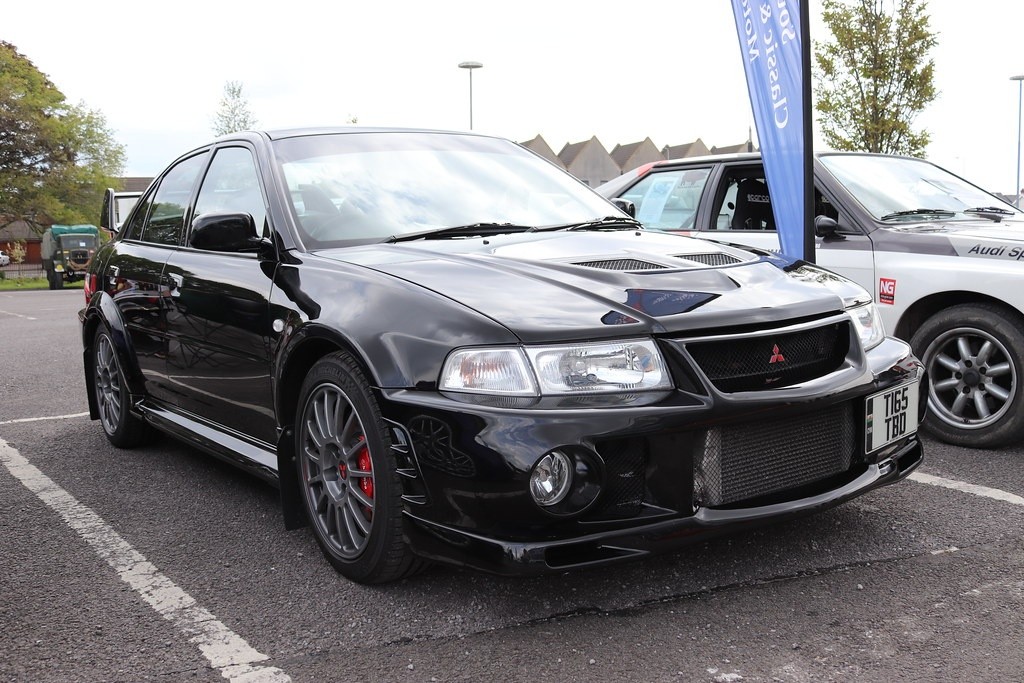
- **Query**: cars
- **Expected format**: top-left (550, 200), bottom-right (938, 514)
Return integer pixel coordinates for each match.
top-left (0, 250), bottom-right (11, 267)
top-left (581, 152), bottom-right (1024, 449)
top-left (75, 124), bottom-right (925, 587)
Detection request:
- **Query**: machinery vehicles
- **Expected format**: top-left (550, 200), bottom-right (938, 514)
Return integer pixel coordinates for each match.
top-left (40, 224), bottom-right (103, 290)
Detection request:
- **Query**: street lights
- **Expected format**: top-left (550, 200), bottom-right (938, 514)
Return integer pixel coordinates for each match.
top-left (458, 61), bottom-right (484, 133)
top-left (1008, 75), bottom-right (1024, 206)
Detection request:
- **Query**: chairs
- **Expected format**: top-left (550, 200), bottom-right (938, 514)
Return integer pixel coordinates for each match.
top-left (729, 178), bottom-right (770, 230)
